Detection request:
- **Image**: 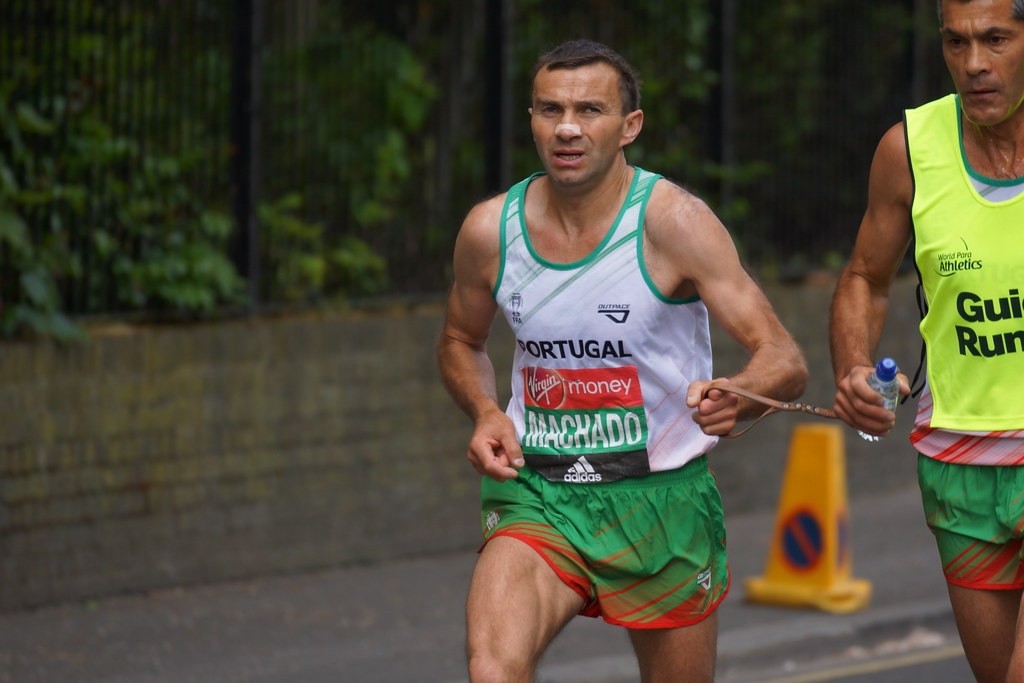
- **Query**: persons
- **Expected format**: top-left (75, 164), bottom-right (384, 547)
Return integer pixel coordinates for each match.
top-left (435, 40), bottom-right (808, 683)
top-left (826, 0), bottom-right (1024, 683)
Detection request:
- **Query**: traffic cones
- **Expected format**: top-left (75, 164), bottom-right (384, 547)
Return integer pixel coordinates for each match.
top-left (744, 423), bottom-right (870, 614)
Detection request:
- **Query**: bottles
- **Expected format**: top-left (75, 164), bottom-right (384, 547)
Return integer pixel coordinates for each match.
top-left (856, 358), bottom-right (899, 442)
